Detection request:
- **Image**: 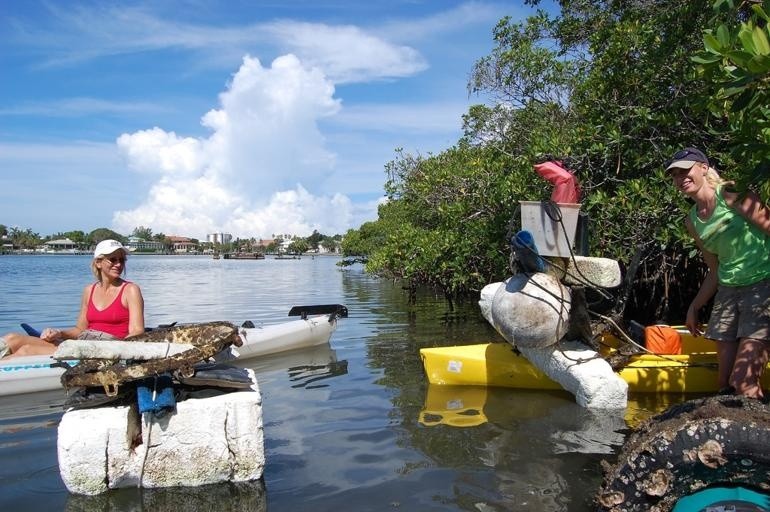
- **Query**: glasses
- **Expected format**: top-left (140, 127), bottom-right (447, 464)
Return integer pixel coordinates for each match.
top-left (95, 258), bottom-right (127, 263)
top-left (663, 150), bottom-right (696, 170)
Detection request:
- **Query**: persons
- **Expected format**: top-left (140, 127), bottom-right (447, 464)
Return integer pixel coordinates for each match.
top-left (0, 239), bottom-right (144, 362)
top-left (665, 145), bottom-right (770, 398)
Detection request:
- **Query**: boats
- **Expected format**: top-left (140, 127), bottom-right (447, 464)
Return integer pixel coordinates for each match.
top-left (417, 322), bottom-right (737, 396)
top-left (415, 386), bottom-right (697, 434)
top-left (213, 246), bottom-right (301, 260)
top-left (0, 302), bottom-right (349, 396)
top-left (1, 339), bottom-right (346, 443)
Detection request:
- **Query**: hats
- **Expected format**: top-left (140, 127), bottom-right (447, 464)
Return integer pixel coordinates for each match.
top-left (94, 239), bottom-right (130, 258)
top-left (664, 147), bottom-right (709, 178)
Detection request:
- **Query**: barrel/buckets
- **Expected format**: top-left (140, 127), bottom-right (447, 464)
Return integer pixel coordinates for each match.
top-left (519, 200), bottom-right (581, 259)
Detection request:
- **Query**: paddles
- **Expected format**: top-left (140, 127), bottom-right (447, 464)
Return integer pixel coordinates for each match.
top-left (21, 322), bottom-right (41, 337)
